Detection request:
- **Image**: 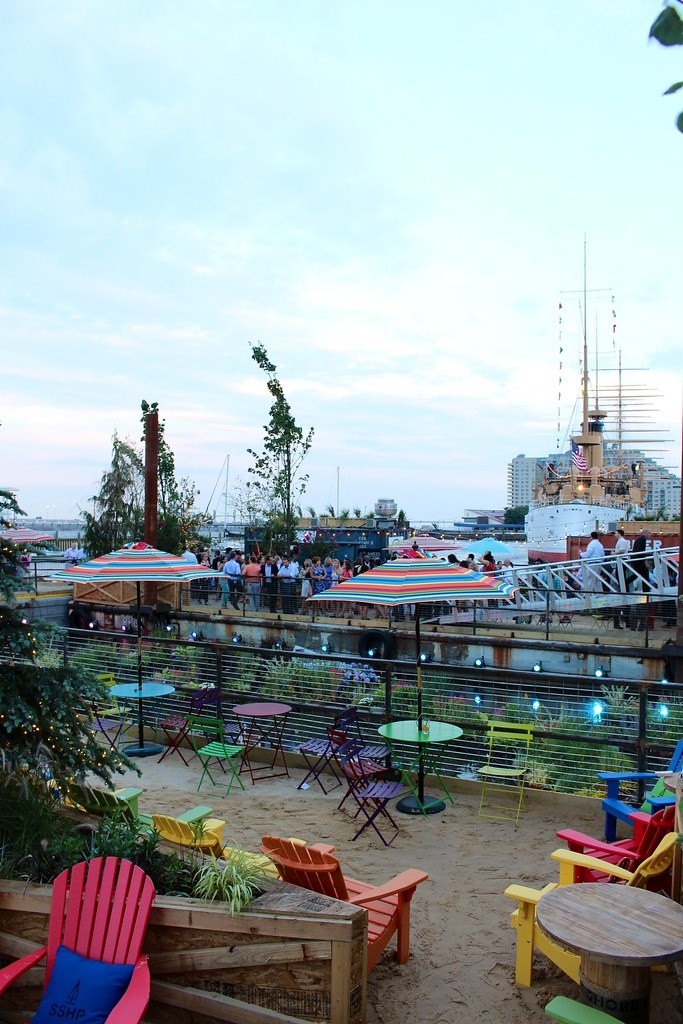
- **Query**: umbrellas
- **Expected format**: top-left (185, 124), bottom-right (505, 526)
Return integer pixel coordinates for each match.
top-left (304, 541), bottom-right (517, 804)
top-left (0, 525), bottom-right (53, 548)
top-left (43, 536), bottom-right (229, 749)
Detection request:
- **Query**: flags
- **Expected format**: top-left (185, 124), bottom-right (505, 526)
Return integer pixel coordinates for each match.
top-left (572, 438), bottom-right (588, 472)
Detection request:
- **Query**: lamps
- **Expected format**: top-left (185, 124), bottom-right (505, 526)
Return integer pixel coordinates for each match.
top-left (534, 661), bottom-right (542, 672)
top-left (322, 643), bottom-right (330, 654)
top-left (167, 624), bottom-right (175, 632)
top-left (420, 651), bottom-right (431, 662)
top-left (631, 463), bottom-right (639, 478)
top-left (368, 647), bottom-right (378, 656)
top-left (474, 657), bottom-right (484, 667)
top-left (232, 636), bottom-right (241, 643)
top-left (193, 630), bottom-right (202, 639)
top-left (595, 666), bottom-right (607, 678)
top-left (90, 619), bottom-right (97, 629)
top-left (121, 624), bottom-right (128, 631)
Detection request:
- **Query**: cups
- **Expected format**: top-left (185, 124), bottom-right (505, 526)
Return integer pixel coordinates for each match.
top-left (423, 716), bottom-right (431, 735)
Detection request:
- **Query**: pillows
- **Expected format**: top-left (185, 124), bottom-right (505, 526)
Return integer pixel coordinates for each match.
top-left (29, 947), bottom-right (135, 1024)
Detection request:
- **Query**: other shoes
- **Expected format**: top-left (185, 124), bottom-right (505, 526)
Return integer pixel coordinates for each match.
top-left (205, 601), bottom-right (208, 605)
top-left (197, 600), bottom-right (202, 604)
top-left (221, 603), bottom-right (389, 619)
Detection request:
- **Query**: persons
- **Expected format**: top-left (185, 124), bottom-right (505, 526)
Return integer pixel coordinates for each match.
top-left (535, 558), bottom-right (582, 622)
top-left (611, 529), bottom-right (630, 592)
top-left (20, 552), bottom-right (31, 584)
top-left (63, 543), bottom-right (88, 585)
top-left (602, 561), bottom-right (680, 630)
top-left (579, 532), bottom-right (604, 598)
top-left (181, 540), bottom-right (514, 621)
top-left (626, 528), bottom-right (651, 592)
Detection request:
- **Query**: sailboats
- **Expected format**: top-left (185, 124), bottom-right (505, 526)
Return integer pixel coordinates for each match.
top-left (523, 243), bottom-right (650, 566)
top-left (196, 454), bottom-right (244, 551)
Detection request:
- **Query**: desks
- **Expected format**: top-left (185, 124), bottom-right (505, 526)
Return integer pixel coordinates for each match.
top-left (108, 682), bottom-right (176, 757)
top-left (232, 702), bottom-right (292, 786)
top-left (536, 882), bottom-right (683, 1024)
top-left (378, 720), bottom-right (464, 816)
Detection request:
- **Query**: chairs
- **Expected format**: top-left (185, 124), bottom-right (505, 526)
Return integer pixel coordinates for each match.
top-left (45, 673), bottom-right (533, 978)
top-left (513, 605), bottom-right (630, 629)
top-left (502, 736), bottom-right (683, 1024)
top-left (0, 856), bottom-right (156, 1024)
